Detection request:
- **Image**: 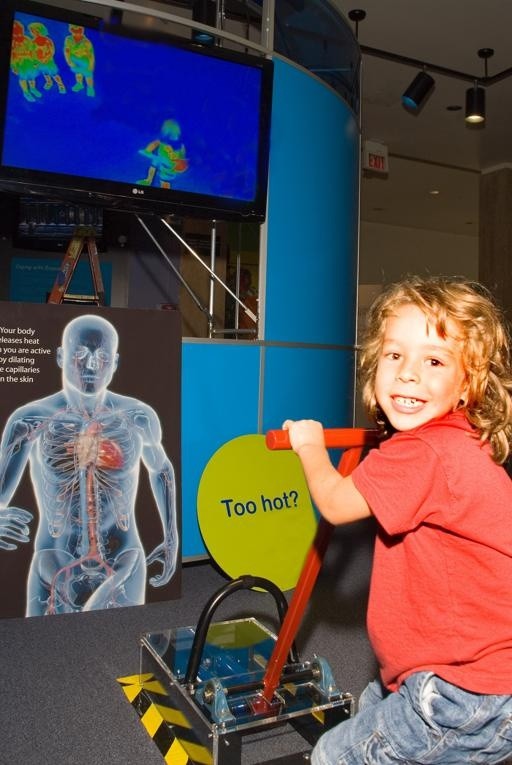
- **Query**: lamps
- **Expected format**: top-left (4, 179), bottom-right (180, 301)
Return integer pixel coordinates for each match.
top-left (402, 65), bottom-right (494, 124)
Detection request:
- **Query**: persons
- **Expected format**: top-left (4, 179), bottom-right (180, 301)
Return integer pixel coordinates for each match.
top-left (134, 118), bottom-right (189, 193)
top-left (282, 273), bottom-right (512, 765)
top-left (7, 15), bottom-right (100, 101)
top-left (0, 313), bottom-right (184, 619)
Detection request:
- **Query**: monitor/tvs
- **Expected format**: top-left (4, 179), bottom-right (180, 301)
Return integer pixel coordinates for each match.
top-left (0, 0), bottom-right (275, 224)
